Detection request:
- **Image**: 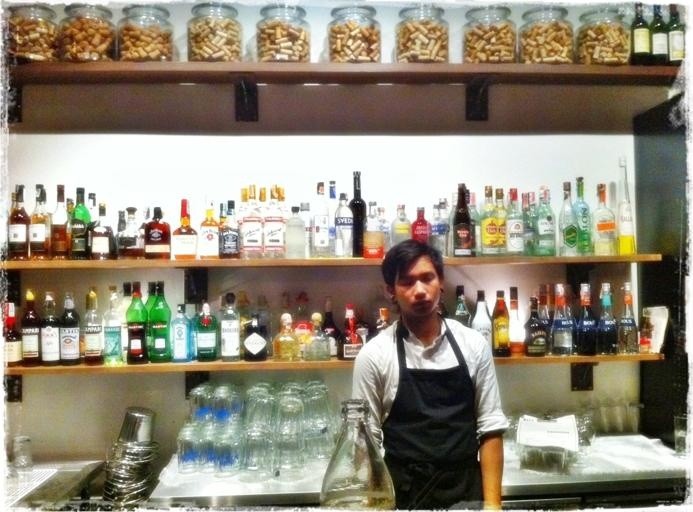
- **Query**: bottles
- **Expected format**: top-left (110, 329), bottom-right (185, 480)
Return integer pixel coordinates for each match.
top-left (116, 4), bottom-right (175, 60)
top-left (394, 6), bottom-right (449, 62)
top-left (576, 10), bottom-right (630, 64)
top-left (462, 6), bottom-right (517, 63)
top-left (630, 2), bottom-right (685, 65)
top-left (327, 6), bottom-right (382, 62)
top-left (187, 2), bottom-right (243, 62)
top-left (2, 280), bottom-right (653, 367)
top-left (11, 404), bottom-right (34, 472)
top-left (519, 7), bottom-right (575, 64)
top-left (58, 3), bottom-right (118, 61)
top-left (319, 399), bottom-right (395, 509)
top-left (7, 3), bottom-right (57, 63)
top-left (257, 3), bottom-right (310, 62)
top-left (9, 157), bottom-right (637, 261)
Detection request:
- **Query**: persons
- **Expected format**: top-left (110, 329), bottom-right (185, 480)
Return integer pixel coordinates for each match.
top-left (349, 239), bottom-right (509, 510)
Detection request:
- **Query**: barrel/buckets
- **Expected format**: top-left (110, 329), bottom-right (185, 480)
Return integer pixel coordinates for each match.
top-left (104, 408), bottom-right (163, 504)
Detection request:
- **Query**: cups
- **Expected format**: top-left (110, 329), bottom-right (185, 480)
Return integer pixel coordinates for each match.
top-left (176, 373), bottom-right (337, 482)
top-left (101, 407), bottom-right (160, 501)
top-left (506, 404), bottom-right (597, 473)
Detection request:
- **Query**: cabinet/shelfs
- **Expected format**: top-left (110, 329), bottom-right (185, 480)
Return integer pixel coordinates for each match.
top-left (3, 61), bottom-right (688, 403)
